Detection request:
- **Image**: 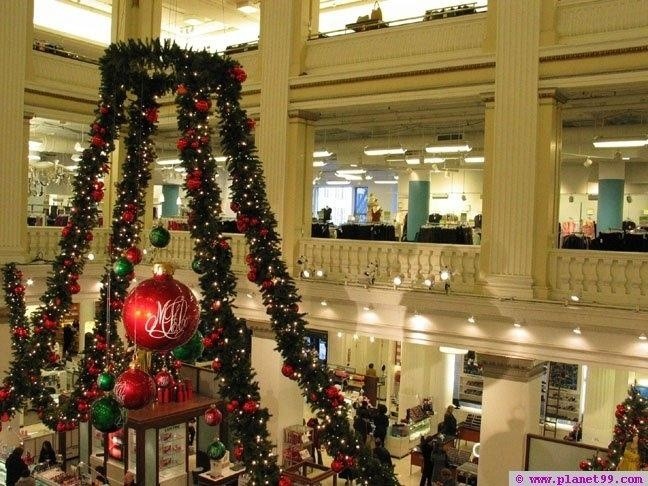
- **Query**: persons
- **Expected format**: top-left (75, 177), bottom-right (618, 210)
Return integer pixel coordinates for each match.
top-left (435, 467), bottom-right (457, 486)
top-left (419, 436), bottom-right (435, 486)
top-left (94, 466), bottom-right (108, 484)
top-left (14, 467), bottom-right (37, 486)
top-left (467, 354), bottom-right (479, 367)
top-left (37, 440), bottom-right (55, 466)
top-left (352, 411), bottom-right (372, 449)
top-left (370, 435), bottom-right (392, 469)
top-left (4, 445), bottom-right (30, 486)
top-left (365, 362), bottom-right (376, 376)
top-left (356, 401), bottom-right (371, 419)
top-left (373, 402), bottom-right (389, 449)
top-left (442, 404), bottom-right (459, 438)
top-left (429, 441), bottom-right (450, 486)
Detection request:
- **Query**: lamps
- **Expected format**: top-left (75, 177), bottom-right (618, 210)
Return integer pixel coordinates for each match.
top-left (29, 116), bottom-right (111, 185)
top-left (154, 149), bottom-right (240, 185)
top-left (312, 123), bottom-right (484, 183)
top-left (236, 0), bottom-right (260, 13)
top-left (578, 126), bottom-right (647, 168)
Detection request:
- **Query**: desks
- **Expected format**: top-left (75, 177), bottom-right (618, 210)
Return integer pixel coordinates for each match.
top-left (197, 466), bottom-right (247, 486)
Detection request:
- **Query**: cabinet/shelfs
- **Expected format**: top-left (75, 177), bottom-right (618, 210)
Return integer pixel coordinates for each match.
top-left (281, 425), bottom-right (315, 474)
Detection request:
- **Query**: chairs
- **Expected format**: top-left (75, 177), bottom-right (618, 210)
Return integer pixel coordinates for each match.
top-left (559, 219), bottom-right (648, 252)
top-left (155, 216), bottom-right (242, 233)
top-left (24, 214), bottom-right (103, 227)
top-left (309, 216), bottom-right (481, 245)
top-left (409, 449), bottom-right (424, 475)
